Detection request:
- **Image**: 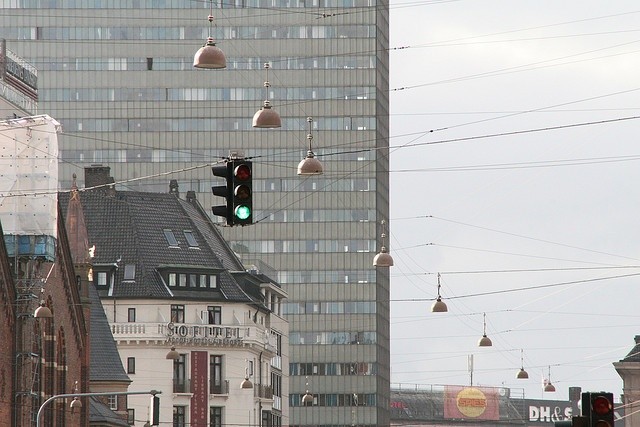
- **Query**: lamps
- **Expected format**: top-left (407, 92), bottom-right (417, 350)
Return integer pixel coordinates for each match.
top-left (240, 358), bottom-right (253, 388)
top-left (517, 348), bottom-right (528, 378)
top-left (252, 61), bottom-right (282, 129)
top-left (192, 0), bottom-right (227, 70)
top-left (33, 278), bottom-right (53, 318)
top-left (432, 272), bottom-right (448, 312)
top-left (297, 116), bottom-right (325, 176)
top-left (303, 375), bottom-right (314, 403)
top-left (544, 364), bottom-right (555, 391)
top-left (479, 311), bottom-right (492, 346)
top-left (165, 344), bottom-right (180, 360)
top-left (372, 219), bottom-right (394, 267)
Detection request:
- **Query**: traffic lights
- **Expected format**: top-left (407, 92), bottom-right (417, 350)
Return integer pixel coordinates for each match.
top-left (212, 159), bottom-right (252, 227)
top-left (576, 390), bottom-right (615, 427)
